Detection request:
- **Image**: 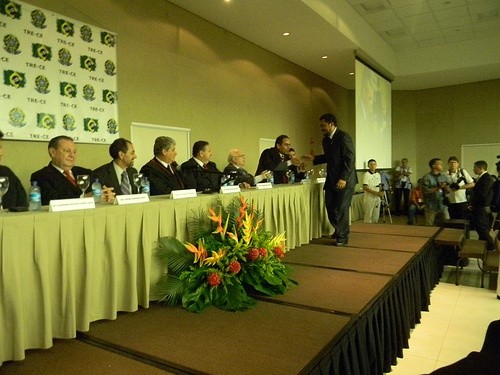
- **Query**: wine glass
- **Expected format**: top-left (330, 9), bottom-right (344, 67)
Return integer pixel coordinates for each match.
top-left (77, 174), bottom-right (90, 198)
top-left (0, 176), bottom-right (9, 212)
top-left (133, 173), bottom-right (144, 194)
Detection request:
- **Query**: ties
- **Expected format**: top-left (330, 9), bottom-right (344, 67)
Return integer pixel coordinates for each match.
top-left (64, 170), bottom-right (78, 187)
top-left (168, 165), bottom-right (173, 174)
top-left (203, 163), bottom-right (208, 170)
top-left (120, 172), bottom-right (129, 194)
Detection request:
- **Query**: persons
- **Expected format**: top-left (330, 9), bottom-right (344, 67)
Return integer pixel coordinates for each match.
top-left (255, 135), bottom-right (302, 183)
top-left (408, 179), bottom-right (425, 225)
top-left (30, 136), bottom-right (114, 206)
top-left (223, 149), bottom-right (272, 186)
top-left (181, 140), bottom-right (250, 195)
top-left (0, 130), bottom-right (27, 211)
top-left (363, 159), bottom-right (383, 224)
top-left (422, 157), bottom-right (450, 227)
top-left (139, 136), bottom-right (187, 195)
top-left (288, 148), bottom-right (306, 182)
top-left (392, 158), bottom-right (412, 217)
top-left (92, 138), bottom-right (142, 194)
top-left (472, 160), bottom-right (494, 251)
top-left (490, 180), bottom-right (500, 241)
top-left (445, 156), bottom-right (475, 219)
top-left (301, 114), bottom-right (358, 245)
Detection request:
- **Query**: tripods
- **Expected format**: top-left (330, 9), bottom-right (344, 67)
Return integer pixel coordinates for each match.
top-left (376, 184), bottom-right (393, 225)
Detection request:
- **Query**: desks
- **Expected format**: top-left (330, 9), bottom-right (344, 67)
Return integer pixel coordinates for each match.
top-left (0, 179), bottom-right (364, 367)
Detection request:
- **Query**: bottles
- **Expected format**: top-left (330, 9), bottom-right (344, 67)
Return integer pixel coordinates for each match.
top-left (141, 177), bottom-right (150, 198)
top-left (287, 170), bottom-right (293, 184)
top-left (91, 178), bottom-right (102, 202)
top-left (267, 173), bottom-right (274, 187)
top-left (221, 174), bottom-right (227, 186)
top-left (28, 181), bottom-right (42, 212)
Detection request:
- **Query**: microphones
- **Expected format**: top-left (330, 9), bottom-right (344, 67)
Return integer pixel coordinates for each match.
top-left (178, 164), bottom-right (199, 170)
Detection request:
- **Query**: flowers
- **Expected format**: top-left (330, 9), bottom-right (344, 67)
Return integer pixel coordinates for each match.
top-left (151, 191), bottom-right (299, 314)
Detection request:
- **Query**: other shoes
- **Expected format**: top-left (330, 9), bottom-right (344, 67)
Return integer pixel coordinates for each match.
top-left (334, 240), bottom-right (348, 246)
